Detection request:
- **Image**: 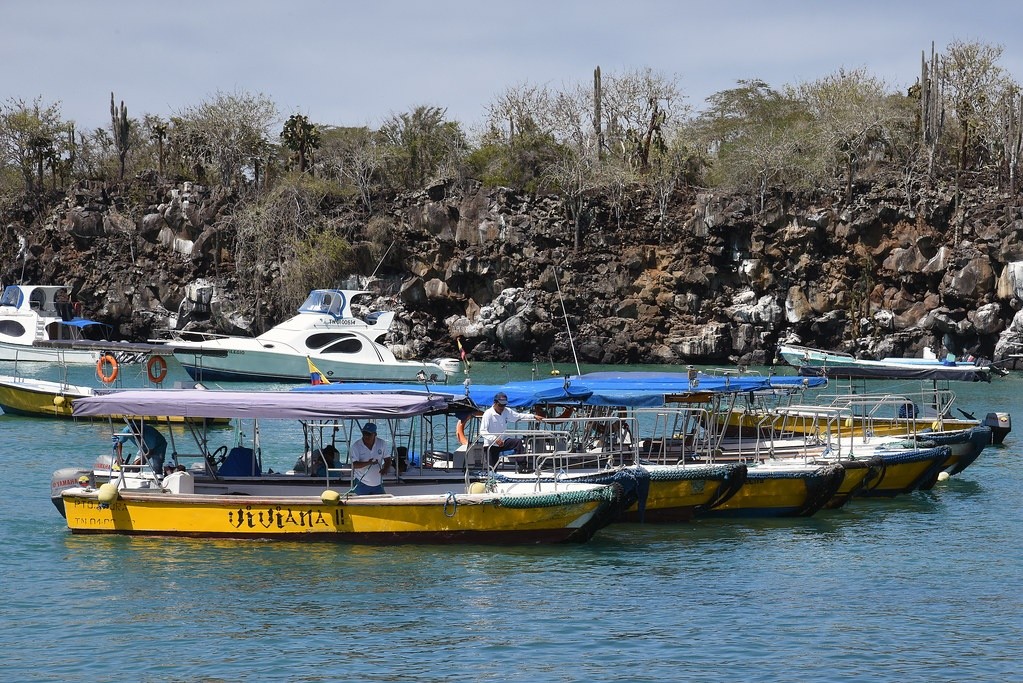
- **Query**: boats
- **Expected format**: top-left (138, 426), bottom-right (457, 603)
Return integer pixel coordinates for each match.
top-left (0, 339), bottom-right (1012, 545)
top-left (0, 249), bottom-right (116, 365)
top-left (154, 235), bottom-right (462, 385)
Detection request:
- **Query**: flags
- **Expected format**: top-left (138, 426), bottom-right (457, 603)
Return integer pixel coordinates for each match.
top-left (307, 358), bottom-right (330, 385)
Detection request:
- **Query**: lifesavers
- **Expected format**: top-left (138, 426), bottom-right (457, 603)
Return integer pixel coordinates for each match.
top-left (97, 355), bottom-right (118, 383)
top-left (147, 355), bottom-right (167, 383)
top-left (74, 302), bottom-right (81, 316)
top-left (456, 411), bottom-right (485, 445)
top-left (584, 405), bottom-right (628, 434)
top-left (535, 404), bottom-right (574, 425)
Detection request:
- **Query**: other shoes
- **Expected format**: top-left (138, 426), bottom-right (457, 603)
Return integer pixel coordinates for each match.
top-left (518, 466), bottom-right (532, 473)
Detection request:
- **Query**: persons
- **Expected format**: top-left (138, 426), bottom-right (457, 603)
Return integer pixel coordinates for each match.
top-left (479, 392), bottom-right (546, 473)
top-left (899, 399), bottom-right (919, 418)
top-left (350, 423), bottom-right (391, 496)
top-left (315, 448), bottom-right (341, 476)
top-left (114, 422), bottom-right (168, 474)
top-left (392, 457), bottom-right (418, 473)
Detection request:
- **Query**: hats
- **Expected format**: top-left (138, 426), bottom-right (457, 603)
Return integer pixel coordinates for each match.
top-left (361, 423), bottom-right (377, 433)
top-left (494, 392), bottom-right (508, 405)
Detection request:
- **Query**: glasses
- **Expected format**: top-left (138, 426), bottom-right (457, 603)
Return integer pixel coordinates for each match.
top-left (363, 432), bottom-right (375, 436)
top-left (496, 403), bottom-right (507, 407)
